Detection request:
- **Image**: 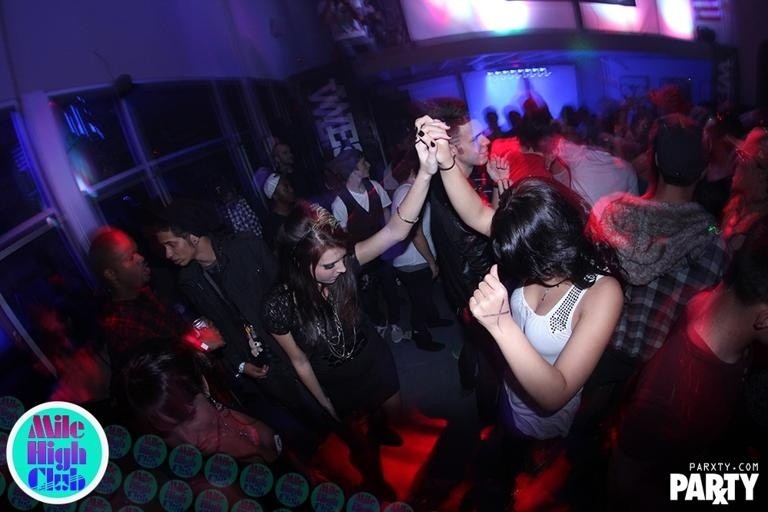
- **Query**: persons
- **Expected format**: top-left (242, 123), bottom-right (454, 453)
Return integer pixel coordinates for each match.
top-left (53, 82), bottom-right (768, 511)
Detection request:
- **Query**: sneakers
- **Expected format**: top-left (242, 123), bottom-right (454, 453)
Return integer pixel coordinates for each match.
top-left (390, 324), bottom-right (402, 343)
top-left (430, 319), bottom-right (453, 328)
top-left (424, 343), bottom-right (443, 352)
top-left (376, 324), bottom-right (387, 338)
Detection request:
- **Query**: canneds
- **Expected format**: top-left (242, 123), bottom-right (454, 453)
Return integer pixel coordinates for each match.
top-left (192, 317), bottom-right (210, 337)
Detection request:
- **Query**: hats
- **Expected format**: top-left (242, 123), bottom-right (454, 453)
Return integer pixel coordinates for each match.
top-left (264, 172), bottom-right (280, 198)
top-left (727, 127), bottom-right (768, 162)
